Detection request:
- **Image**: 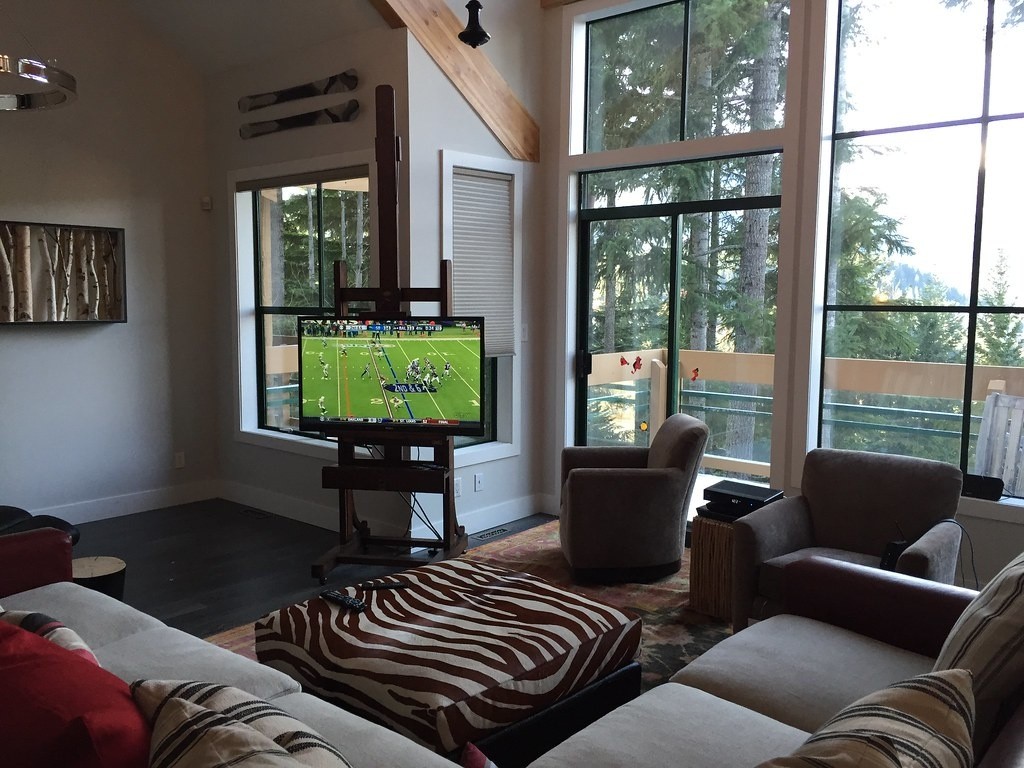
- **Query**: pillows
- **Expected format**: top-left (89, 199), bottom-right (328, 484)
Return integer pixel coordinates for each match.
top-left (756, 668), bottom-right (978, 768)
top-left (130, 680), bottom-right (354, 768)
top-left (0, 618), bottom-right (153, 768)
top-left (934, 551), bottom-right (1024, 763)
top-left (0, 610), bottom-right (103, 669)
top-left (149, 701), bottom-right (307, 768)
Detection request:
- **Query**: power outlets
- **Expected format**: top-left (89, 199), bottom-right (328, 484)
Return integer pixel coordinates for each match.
top-left (454, 477), bottom-right (462, 498)
top-left (175, 451), bottom-right (186, 469)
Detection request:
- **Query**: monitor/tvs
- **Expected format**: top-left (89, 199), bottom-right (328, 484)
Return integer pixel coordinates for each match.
top-left (297, 315), bottom-right (487, 438)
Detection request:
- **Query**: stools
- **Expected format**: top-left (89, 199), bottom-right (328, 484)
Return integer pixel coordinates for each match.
top-left (70, 556), bottom-right (127, 603)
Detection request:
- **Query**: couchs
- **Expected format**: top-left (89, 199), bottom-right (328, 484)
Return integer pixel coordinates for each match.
top-left (0, 412), bottom-right (1024, 768)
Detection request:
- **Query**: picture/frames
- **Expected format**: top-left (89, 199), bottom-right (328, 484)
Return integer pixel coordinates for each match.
top-left (0, 221), bottom-right (128, 326)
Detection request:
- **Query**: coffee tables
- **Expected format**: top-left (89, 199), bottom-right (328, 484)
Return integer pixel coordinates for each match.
top-left (254, 558), bottom-right (642, 768)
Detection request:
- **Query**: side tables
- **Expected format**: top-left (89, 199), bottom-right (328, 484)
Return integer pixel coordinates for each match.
top-left (687, 515), bottom-right (735, 625)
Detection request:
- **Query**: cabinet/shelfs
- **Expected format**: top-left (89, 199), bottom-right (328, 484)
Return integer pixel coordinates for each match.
top-left (312, 421), bottom-right (482, 577)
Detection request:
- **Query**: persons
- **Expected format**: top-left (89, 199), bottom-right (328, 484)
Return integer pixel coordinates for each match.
top-left (403, 357), bottom-right (451, 390)
top-left (319, 340), bottom-right (348, 381)
top-left (301, 321), bottom-right (443, 338)
top-left (318, 396), bottom-right (328, 415)
top-left (461, 321), bottom-right (477, 334)
top-left (361, 363), bottom-right (372, 379)
top-left (379, 375), bottom-right (386, 390)
top-left (392, 395), bottom-right (402, 412)
top-left (372, 339), bottom-right (382, 360)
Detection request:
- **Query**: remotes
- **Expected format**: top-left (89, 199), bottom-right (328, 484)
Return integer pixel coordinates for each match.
top-left (321, 590), bottom-right (367, 613)
top-left (360, 581), bottom-right (409, 590)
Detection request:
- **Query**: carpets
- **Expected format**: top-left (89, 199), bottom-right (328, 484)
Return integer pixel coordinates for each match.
top-left (202, 519), bottom-right (759, 695)
